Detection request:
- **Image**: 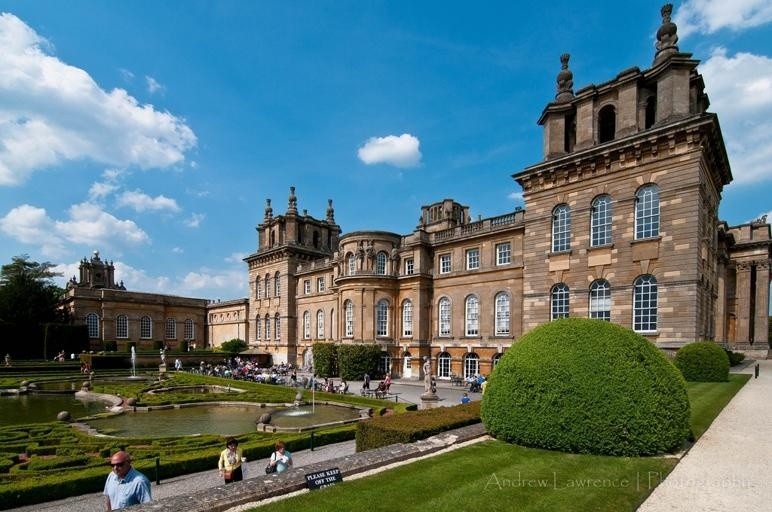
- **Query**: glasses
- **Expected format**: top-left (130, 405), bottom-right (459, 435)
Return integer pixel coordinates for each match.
top-left (111, 461), bottom-right (126, 467)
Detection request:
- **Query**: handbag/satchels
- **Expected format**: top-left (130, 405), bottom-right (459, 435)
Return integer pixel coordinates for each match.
top-left (266, 465), bottom-right (276, 475)
top-left (224, 471), bottom-right (233, 480)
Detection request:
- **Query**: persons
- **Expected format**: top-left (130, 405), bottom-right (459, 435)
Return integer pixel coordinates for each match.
top-left (460, 393), bottom-right (472, 405)
top-left (265, 440), bottom-right (293, 472)
top-left (463, 373), bottom-right (490, 394)
top-left (199, 355), bottom-right (349, 395)
top-left (55, 350), bottom-right (67, 361)
top-left (85, 361), bottom-right (92, 370)
top-left (80, 362), bottom-right (91, 373)
top-left (423, 357), bottom-right (432, 391)
top-left (431, 376), bottom-right (437, 393)
top-left (218, 438), bottom-right (246, 483)
top-left (383, 372), bottom-right (391, 393)
top-left (362, 371), bottom-right (370, 389)
top-left (4, 353), bottom-right (13, 367)
top-left (103, 451), bottom-right (151, 511)
top-left (174, 356), bottom-right (184, 371)
